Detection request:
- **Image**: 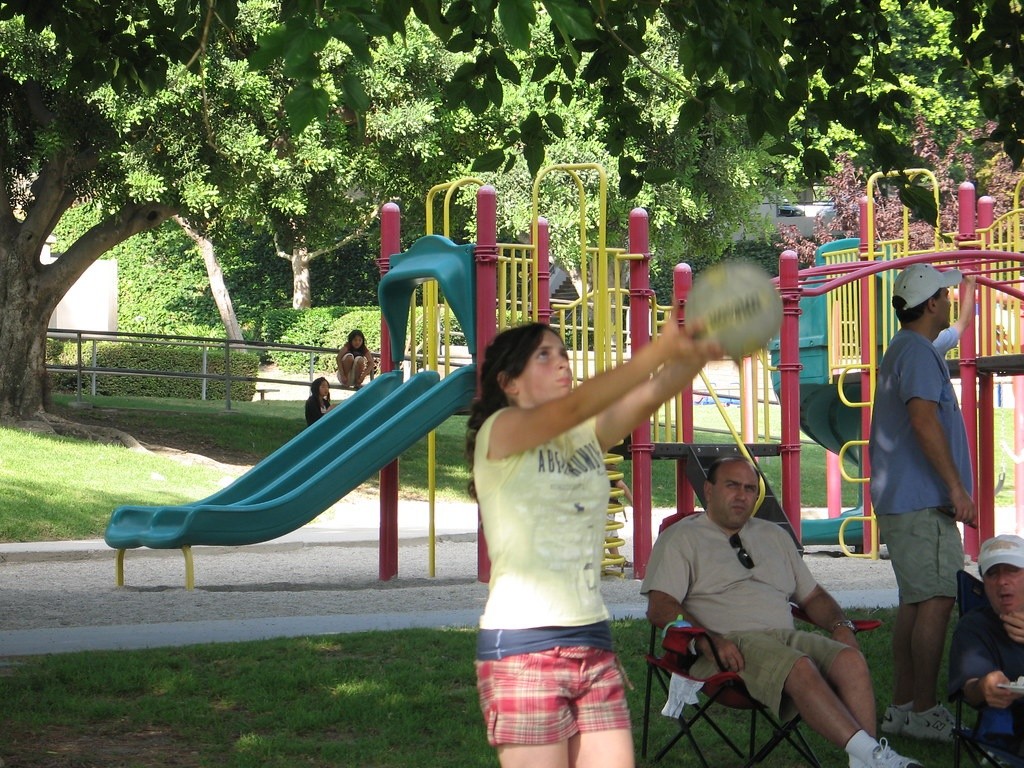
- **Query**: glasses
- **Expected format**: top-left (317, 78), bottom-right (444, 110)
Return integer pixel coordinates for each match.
top-left (729, 533), bottom-right (754, 569)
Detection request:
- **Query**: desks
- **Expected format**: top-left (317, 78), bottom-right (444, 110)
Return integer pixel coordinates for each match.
top-left (255, 388), bottom-right (280, 401)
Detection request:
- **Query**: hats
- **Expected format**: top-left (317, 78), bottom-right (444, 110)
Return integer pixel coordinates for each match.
top-left (977, 535), bottom-right (1024, 576)
top-left (892, 263), bottom-right (963, 310)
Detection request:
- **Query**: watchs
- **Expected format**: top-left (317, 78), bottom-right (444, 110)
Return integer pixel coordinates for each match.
top-left (832, 619), bottom-right (855, 631)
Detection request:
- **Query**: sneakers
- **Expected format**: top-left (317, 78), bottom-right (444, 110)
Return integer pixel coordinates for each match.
top-left (865, 737), bottom-right (924, 768)
top-left (881, 701), bottom-right (913, 735)
top-left (901, 701), bottom-right (971, 743)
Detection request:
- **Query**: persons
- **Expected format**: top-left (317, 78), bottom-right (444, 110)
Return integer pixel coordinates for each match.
top-left (868, 263), bottom-right (980, 744)
top-left (640, 456), bottom-right (922, 768)
top-left (465, 299), bottom-right (725, 768)
top-left (305, 377), bottom-right (334, 426)
top-left (946, 534), bottom-right (1024, 768)
top-left (336, 330), bottom-right (374, 390)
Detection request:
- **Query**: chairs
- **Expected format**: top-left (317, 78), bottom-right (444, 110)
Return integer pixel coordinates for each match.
top-left (951, 569), bottom-right (1024, 768)
top-left (641, 512), bottom-right (881, 768)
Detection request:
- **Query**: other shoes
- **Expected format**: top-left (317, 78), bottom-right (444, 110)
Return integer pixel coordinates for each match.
top-left (354, 384), bottom-right (363, 391)
top-left (344, 383), bottom-right (350, 389)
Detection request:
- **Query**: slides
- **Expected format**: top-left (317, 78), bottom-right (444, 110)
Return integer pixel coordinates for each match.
top-left (102, 362), bottom-right (476, 551)
top-left (769, 237), bottom-right (901, 546)
